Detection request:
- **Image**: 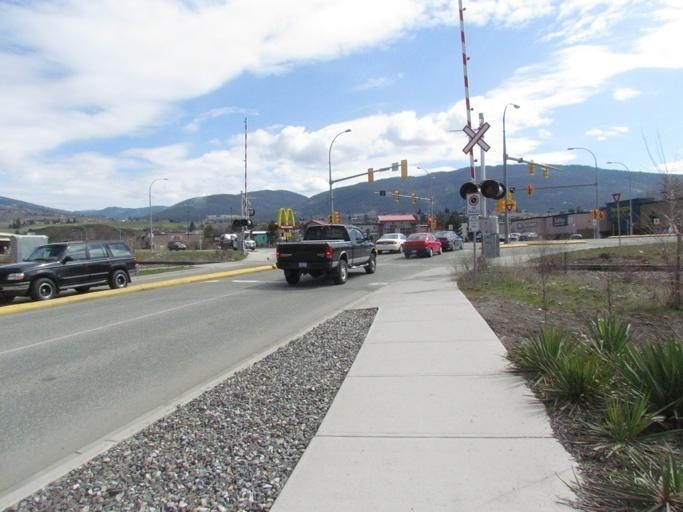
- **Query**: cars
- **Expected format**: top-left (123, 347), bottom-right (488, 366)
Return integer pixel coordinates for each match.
top-left (167, 240), bottom-right (186, 251)
top-left (499, 232), bottom-right (540, 242)
top-left (373, 231), bottom-right (408, 255)
top-left (402, 231), bottom-right (443, 259)
top-left (433, 229), bottom-right (463, 253)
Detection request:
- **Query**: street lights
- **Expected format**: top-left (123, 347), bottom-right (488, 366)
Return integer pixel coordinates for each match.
top-left (148, 177), bottom-right (168, 250)
top-left (566, 146), bottom-right (601, 239)
top-left (327, 128), bottom-right (351, 226)
top-left (607, 161), bottom-right (634, 236)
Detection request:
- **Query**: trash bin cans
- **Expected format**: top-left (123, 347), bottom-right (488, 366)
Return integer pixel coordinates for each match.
top-left (483, 235), bottom-right (500, 258)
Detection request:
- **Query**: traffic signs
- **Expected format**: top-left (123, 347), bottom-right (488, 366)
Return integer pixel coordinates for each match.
top-left (502, 102), bottom-right (522, 241)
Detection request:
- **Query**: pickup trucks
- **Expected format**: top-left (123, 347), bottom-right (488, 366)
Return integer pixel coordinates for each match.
top-left (274, 223), bottom-right (379, 285)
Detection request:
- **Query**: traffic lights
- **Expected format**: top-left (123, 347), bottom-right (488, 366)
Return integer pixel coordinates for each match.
top-left (481, 179), bottom-right (507, 200)
top-left (233, 218), bottom-right (253, 227)
top-left (459, 180), bottom-right (481, 200)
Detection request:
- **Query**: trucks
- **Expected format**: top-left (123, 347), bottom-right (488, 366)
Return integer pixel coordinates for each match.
top-left (214, 233), bottom-right (256, 251)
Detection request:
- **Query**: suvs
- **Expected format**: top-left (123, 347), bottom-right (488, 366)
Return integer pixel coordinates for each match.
top-left (0, 240), bottom-right (138, 308)
